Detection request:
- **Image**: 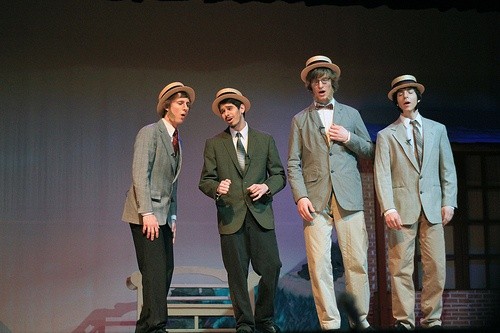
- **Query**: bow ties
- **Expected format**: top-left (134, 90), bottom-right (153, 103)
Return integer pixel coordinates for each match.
top-left (316, 103), bottom-right (334, 110)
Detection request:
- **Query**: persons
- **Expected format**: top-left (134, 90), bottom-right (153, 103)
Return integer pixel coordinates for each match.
top-left (198, 89), bottom-right (288, 333)
top-left (121, 82), bottom-right (195, 333)
top-left (286, 56), bottom-right (374, 333)
top-left (373, 74), bottom-right (458, 332)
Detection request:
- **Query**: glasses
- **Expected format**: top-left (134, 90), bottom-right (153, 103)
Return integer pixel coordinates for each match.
top-left (311, 77), bottom-right (331, 85)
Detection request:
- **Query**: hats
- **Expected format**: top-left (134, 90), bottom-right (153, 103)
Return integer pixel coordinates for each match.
top-left (211, 87), bottom-right (251, 118)
top-left (156, 81), bottom-right (196, 117)
top-left (388, 75), bottom-right (425, 102)
top-left (301, 55), bottom-right (341, 85)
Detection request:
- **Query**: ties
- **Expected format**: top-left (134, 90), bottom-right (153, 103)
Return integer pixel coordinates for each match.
top-left (173, 129), bottom-right (179, 155)
top-left (410, 120), bottom-right (423, 169)
top-left (236, 133), bottom-right (246, 172)
